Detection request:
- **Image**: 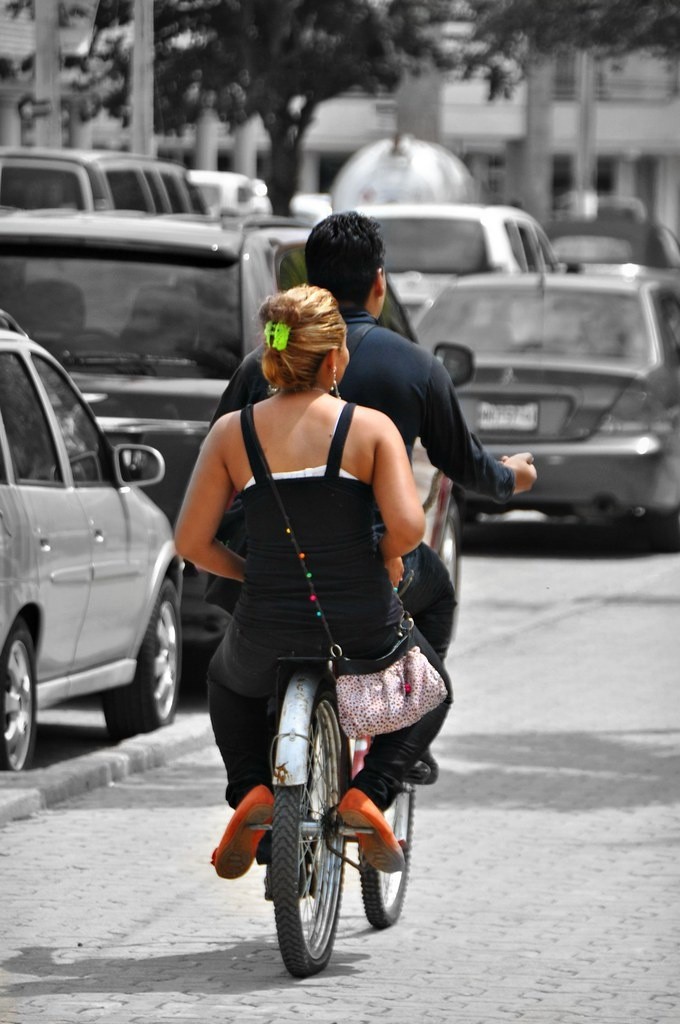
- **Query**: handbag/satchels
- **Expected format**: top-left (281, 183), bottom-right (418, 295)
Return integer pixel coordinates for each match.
top-left (331, 631), bottom-right (448, 739)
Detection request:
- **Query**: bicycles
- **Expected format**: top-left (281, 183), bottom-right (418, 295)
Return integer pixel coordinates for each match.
top-left (242, 468), bottom-right (455, 980)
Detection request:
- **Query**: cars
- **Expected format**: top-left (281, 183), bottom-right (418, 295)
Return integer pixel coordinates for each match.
top-left (541, 216), bottom-right (680, 274)
top-left (413, 268), bottom-right (679, 553)
top-left (0, 307), bottom-right (186, 774)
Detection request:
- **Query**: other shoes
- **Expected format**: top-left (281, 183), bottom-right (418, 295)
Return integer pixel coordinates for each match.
top-left (338, 787), bottom-right (406, 873)
top-left (264, 862), bottom-right (306, 901)
top-left (210, 784), bottom-right (274, 879)
top-left (415, 749), bottom-right (441, 786)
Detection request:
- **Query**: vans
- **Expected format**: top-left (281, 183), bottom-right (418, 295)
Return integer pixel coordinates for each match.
top-left (0, 140), bottom-right (212, 214)
top-left (348, 202), bottom-right (583, 326)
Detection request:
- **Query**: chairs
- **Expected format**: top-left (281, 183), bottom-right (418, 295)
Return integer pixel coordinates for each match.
top-left (18, 279), bottom-right (116, 352)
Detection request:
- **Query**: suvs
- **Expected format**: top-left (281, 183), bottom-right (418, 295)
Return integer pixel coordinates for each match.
top-left (0, 200), bottom-right (477, 693)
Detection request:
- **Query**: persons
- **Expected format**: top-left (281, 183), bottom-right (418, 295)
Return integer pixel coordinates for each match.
top-left (207, 212), bottom-right (536, 786)
top-left (174, 283), bottom-right (426, 879)
